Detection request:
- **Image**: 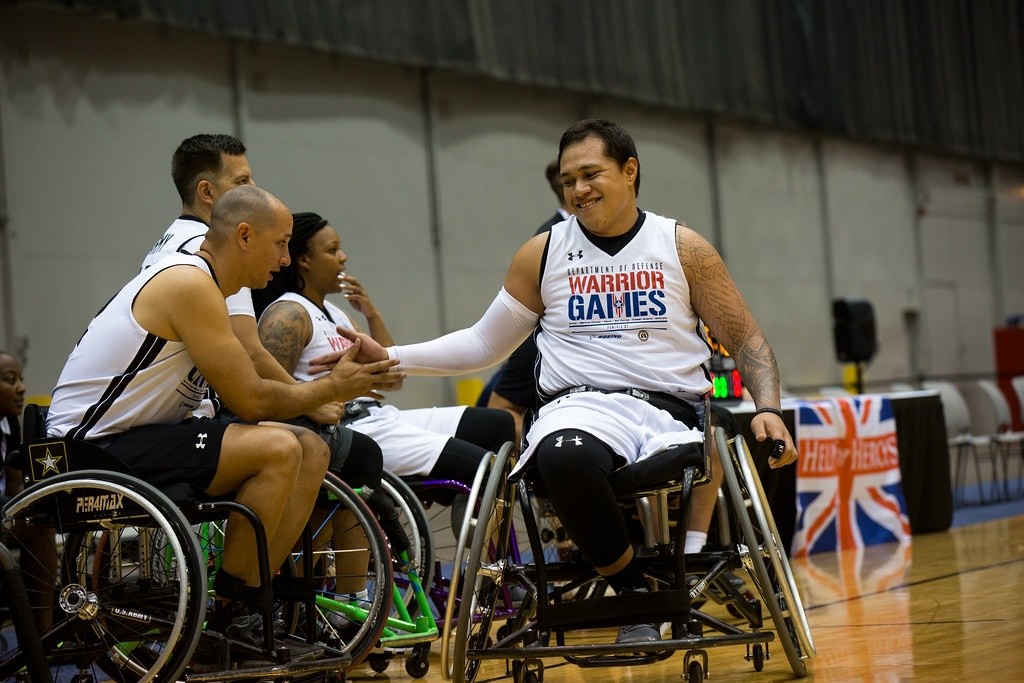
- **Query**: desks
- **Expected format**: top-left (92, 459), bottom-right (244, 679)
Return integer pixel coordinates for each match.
top-left (790, 529), bottom-right (965, 683)
top-left (705, 390), bottom-right (956, 556)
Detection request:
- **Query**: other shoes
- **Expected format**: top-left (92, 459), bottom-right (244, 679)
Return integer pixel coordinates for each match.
top-left (496, 583), bottom-right (528, 601)
top-left (207, 598), bottom-right (274, 648)
top-left (331, 589), bottom-right (411, 636)
top-left (695, 570), bottom-right (747, 592)
top-left (613, 585), bottom-right (662, 656)
top-left (284, 597), bottom-right (339, 648)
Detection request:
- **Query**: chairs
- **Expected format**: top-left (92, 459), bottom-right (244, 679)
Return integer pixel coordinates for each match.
top-left (819, 377), bottom-right (1024, 508)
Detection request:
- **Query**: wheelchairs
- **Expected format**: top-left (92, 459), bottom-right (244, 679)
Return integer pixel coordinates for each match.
top-left (0, 391), bottom-right (549, 683)
top-left (438, 396), bottom-right (818, 683)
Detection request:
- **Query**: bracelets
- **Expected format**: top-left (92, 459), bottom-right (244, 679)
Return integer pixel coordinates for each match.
top-left (754, 408), bottom-right (783, 420)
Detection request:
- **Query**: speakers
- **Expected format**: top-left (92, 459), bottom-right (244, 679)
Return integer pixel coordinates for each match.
top-left (833, 299), bottom-right (877, 363)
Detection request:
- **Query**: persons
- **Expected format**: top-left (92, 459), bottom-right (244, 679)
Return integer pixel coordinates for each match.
top-left (0, 117), bottom-right (799, 683)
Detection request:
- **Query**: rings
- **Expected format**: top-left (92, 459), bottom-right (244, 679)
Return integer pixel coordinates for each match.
top-left (771, 439), bottom-right (785, 459)
